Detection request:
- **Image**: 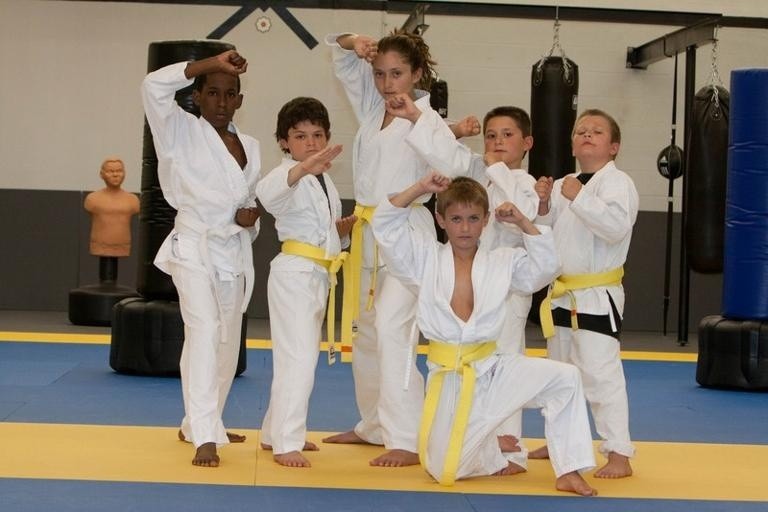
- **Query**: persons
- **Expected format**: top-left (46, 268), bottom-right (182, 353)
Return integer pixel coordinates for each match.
top-left (370, 171), bottom-right (600, 496)
top-left (255, 96), bottom-right (358, 468)
top-left (442, 106), bottom-right (540, 475)
top-left (324, 32), bottom-right (472, 468)
top-left (527, 111), bottom-right (639, 480)
top-left (83, 158), bottom-right (141, 257)
top-left (139, 49), bottom-right (261, 467)
